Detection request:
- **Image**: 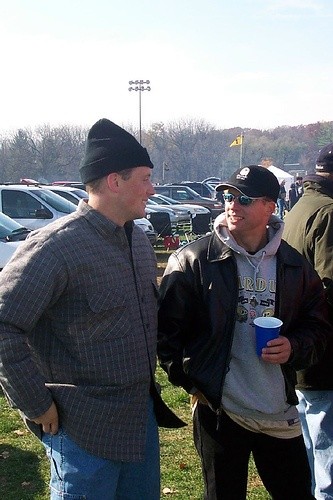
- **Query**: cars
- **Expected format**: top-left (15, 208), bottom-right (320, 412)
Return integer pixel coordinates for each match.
top-left (144, 176), bottom-right (230, 238)
top-left (37, 185), bottom-right (155, 234)
top-left (0, 211), bottom-right (38, 272)
top-left (0, 183), bottom-right (80, 231)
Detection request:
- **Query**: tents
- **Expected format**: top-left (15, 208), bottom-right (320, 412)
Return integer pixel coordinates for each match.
top-left (266, 165), bottom-right (294, 201)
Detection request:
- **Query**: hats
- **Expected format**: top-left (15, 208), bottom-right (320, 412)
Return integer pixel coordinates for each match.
top-left (80, 118), bottom-right (153, 184)
top-left (316, 144), bottom-right (333, 173)
top-left (217, 165), bottom-right (281, 203)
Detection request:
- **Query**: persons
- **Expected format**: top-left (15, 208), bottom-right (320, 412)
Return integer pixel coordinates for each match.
top-left (0, 118), bottom-right (188, 500)
top-left (157, 166), bottom-right (333, 500)
top-left (281, 143), bottom-right (333, 500)
top-left (289, 183), bottom-right (296, 209)
top-left (295, 176), bottom-right (304, 201)
top-left (277, 178), bottom-right (288, 220)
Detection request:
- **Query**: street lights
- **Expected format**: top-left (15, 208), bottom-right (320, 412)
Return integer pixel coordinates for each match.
top-left (128, 79), bottom-right (152, 145)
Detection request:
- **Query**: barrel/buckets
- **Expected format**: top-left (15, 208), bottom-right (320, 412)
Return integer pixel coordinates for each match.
top-left (192, 213), bottom-right (210, 235)
top-left (163, 234), bottom-right (179, 249)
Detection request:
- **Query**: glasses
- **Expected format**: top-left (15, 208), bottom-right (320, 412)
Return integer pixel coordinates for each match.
top-left (223, 194), bottom-right (256, 205)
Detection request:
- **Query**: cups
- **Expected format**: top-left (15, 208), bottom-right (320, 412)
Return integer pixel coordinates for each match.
top-left (253, 316), bottom-right (283, 358)
